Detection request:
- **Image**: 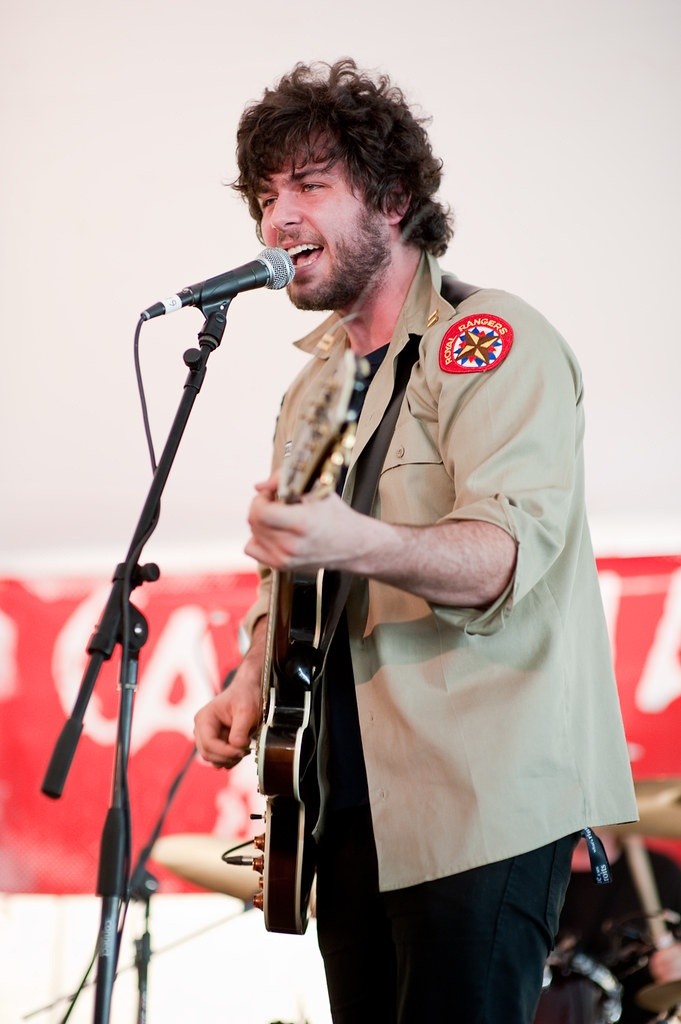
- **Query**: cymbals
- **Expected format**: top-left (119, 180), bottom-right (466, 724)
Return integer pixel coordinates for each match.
top-left (595, 776), bottom-right (681, 838)
top-left (150, 830), bottom-right (260, 902)
top-left (636, 976), bottom-right (681, 1014)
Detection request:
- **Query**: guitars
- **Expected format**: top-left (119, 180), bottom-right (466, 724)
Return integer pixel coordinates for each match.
top-left (250, 347), bottom-right (370, 935)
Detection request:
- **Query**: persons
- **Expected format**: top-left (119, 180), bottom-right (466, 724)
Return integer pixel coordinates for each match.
top-left (196, 60), bottom-right (640, 1024)
top-left (549, 835), bottom-right (681, 1024)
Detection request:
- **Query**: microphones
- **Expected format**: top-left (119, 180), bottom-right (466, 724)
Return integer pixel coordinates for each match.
top-left (140, 248), bottom-right (297, 319)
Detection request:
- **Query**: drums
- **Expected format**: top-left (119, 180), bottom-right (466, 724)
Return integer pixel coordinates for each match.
top-left (530, 950), bottom-right (622, 1024)
